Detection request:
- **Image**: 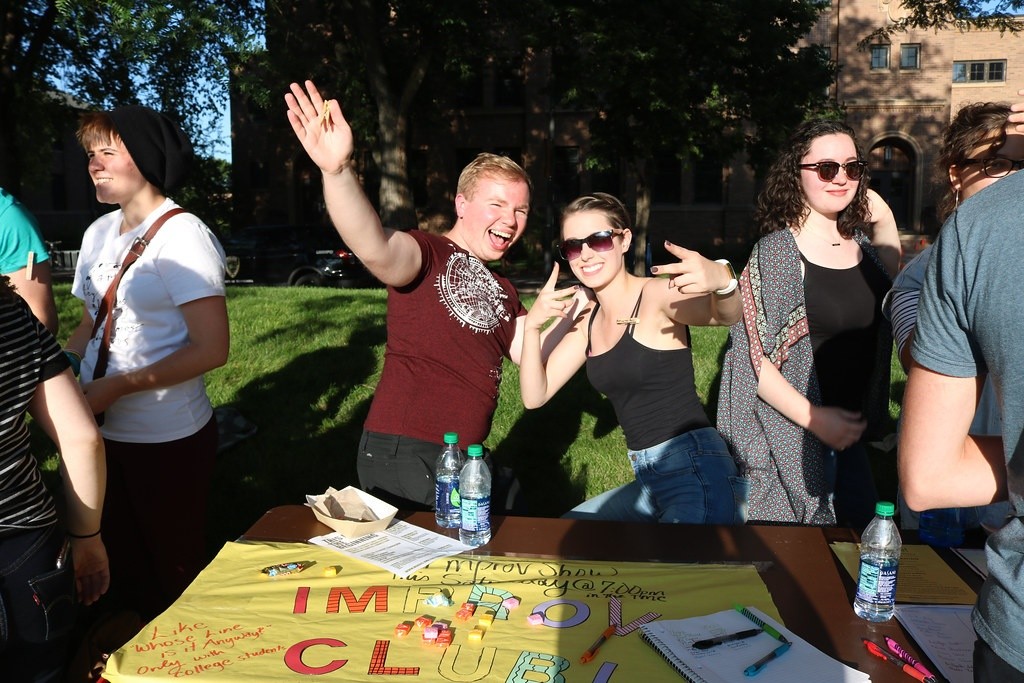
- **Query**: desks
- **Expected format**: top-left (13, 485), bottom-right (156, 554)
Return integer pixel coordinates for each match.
top-left (100, 504), bottom-right (1024, 683)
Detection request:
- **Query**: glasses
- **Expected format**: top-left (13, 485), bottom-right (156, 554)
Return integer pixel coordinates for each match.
top-left (797, 161), bottom-right (869, 182)
top-left (555, 229), bottom-right (623, 260)
top-left (959, 156), bottom-right (1024, 177)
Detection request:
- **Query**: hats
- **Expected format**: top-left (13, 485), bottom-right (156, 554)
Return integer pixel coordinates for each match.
top-left (107, 106), bottom-right (193, 195)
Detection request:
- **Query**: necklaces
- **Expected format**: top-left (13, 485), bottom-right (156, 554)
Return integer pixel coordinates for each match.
top-left (805, 222), bottom-right (840, 246)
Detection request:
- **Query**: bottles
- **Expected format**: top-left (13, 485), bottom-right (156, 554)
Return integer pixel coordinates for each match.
top-left (459, 445), bottom-right (492, 546)
top-left (854, 502), bottom-right (901, 623)
top-left (435, 432), bottom-right (465, 528)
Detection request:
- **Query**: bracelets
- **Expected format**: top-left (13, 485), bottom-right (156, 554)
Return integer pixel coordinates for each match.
top-left (70, 351), bottom-right (81, 360)
top-left (712, 259), bottom-right (738, 295)
top-left (69, 529), bottom-right (101, 538)
top-left (64, 348), bottom-right (83, 357)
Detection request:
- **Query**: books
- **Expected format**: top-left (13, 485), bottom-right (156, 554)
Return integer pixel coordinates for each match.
top-left (639, 605), bottom-right (872, 683)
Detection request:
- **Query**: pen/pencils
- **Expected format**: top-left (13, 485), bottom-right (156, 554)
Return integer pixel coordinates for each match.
top-left (580, 621), bottom-right (619, 665)
top-left (731, 600), bottom-right (790, 644)
top-left (863, 638), bottom-right (933, 683)
top-left (743, 641), bottom-right (792, 677)
top-left (885, 636), bottom-right (938, 683)
top-left (691, 628), bottom-right (765, 652)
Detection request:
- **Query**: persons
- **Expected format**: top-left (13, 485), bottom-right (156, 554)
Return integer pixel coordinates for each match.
top-left (63, 105), bottom-right (229, 651)
top-left (882, 89), bottom-right (1024, 530)
top-left (285, 78), bottom-right (595, 515)
top-left (520, 193), bottom-right (752, 526)
top-left (0, 187), bottom-right (58, 336)
top-left (0, 275), bottom-right (109, 683)
top-left (897, 166), bottom-right (1024, 683)
top-left (718, 120), bottom-right (901, 526)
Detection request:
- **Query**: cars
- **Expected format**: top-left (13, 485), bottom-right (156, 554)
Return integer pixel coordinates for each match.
top-left (224, 223), bottom-right (376, 288)
